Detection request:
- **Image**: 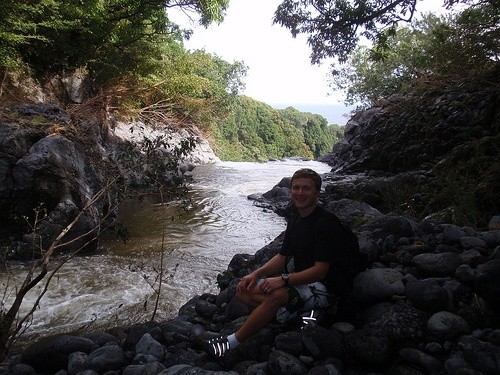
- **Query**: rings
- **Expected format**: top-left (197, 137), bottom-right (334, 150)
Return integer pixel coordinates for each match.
top-left (265, 287), bottom-right (268, 290)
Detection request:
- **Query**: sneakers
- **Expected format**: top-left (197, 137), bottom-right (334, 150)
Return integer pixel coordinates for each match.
top-left (201, 336), bottom-right (235, 363)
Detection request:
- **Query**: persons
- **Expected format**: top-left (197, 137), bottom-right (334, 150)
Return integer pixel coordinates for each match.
top-left (201, 168), bottom-right (341, 362)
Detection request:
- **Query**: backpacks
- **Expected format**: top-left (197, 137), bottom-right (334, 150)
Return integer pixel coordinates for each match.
top-left (314, 212), bottom-right (360, 281)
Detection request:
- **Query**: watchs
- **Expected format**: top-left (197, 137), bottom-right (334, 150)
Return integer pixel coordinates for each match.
top-left (281, 273), bottom-right (289, 288)
